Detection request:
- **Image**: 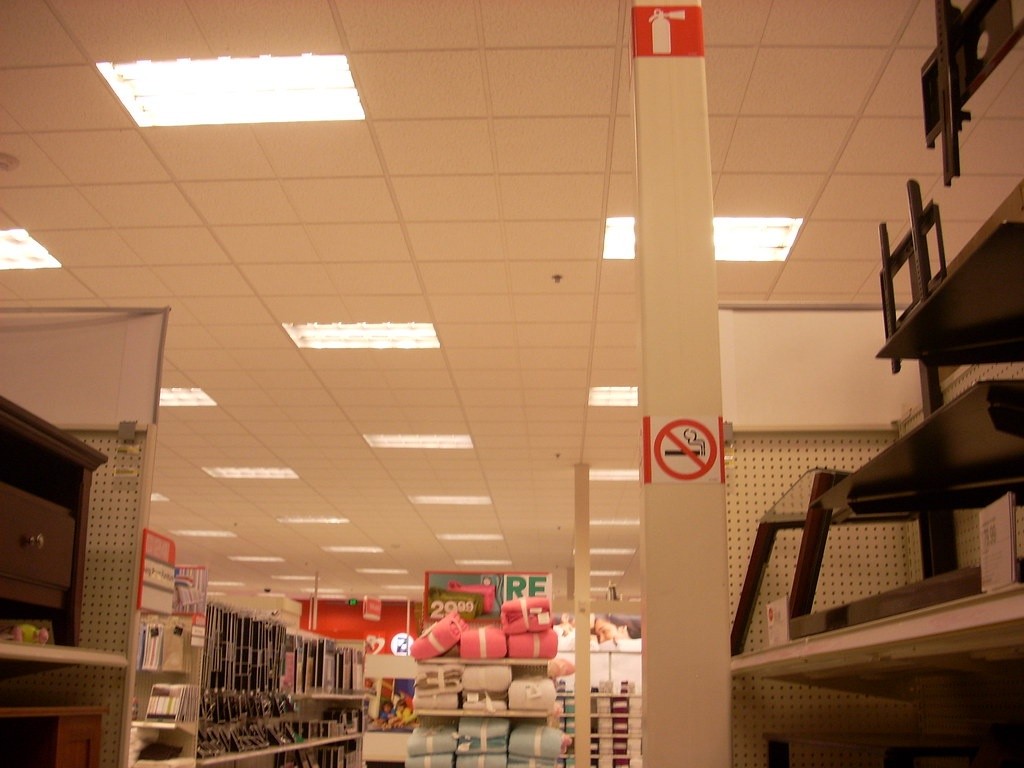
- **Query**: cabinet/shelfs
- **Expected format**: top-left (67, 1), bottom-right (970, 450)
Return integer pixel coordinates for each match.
top-left (557, 693), bottom-right (642, 768)
top-left (1, 420), bottom-right (161, 768)
top-left (0, 396), bottom-right (108, 646)
top-left (414, 652), bottom-right (549, 768)
top-left (134, 610), bottom-right (365, 768)
top-left (128, 720), bottom-right (198, 768)
